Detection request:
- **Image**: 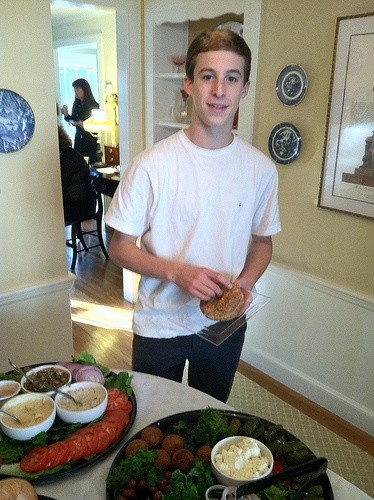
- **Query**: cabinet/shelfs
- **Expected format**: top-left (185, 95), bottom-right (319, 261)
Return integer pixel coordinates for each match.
top-left (157, 8), bottom-right (244, 135)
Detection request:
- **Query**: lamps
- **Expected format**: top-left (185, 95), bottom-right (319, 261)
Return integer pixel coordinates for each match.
top-left (83, 109), bottom-right (113, 143)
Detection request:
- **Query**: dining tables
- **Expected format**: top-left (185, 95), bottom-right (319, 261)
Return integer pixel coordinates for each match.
top-left (32, 368), bottom-right (374, 500)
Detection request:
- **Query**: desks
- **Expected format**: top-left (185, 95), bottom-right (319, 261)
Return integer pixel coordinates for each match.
top-left (89, 167), bottom-right (120, 198)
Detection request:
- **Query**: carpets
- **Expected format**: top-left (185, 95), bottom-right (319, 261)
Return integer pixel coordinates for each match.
top-left (181, 360), bottom-right (374, 500)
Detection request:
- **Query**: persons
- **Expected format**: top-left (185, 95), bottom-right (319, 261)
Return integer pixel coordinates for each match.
top-left (58, 126), bottom-right (97, 225)
top-left (59, 79), bottom-right (100, 164)
top-left (102, 29), bottom-right (282, 404)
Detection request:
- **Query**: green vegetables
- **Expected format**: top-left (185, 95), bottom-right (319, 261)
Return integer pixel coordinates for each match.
top-left (72, 352), bottom-right (133, 397)
top-left (0, 414), bottom-right (98, 478)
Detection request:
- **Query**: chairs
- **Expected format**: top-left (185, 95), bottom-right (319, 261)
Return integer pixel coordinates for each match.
top-left (104, 145), bottom-right (119, 166)
top-left (64, 168), bottom-right (109, 271)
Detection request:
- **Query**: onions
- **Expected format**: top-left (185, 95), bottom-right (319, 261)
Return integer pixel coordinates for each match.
top-left (52, 361), bottom-right (105, 387)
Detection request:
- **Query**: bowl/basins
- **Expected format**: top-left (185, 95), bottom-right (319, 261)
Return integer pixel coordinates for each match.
top-left (210, 436), bottom-right (274, 486)
top-left (0, 380), bottom-right (20, 401)
top-left (21, 364), bottom-right (72, 395)
top-left (0, 393), bottom-right (56, 441)
top-left (54, 381), bottom-right (108, 424)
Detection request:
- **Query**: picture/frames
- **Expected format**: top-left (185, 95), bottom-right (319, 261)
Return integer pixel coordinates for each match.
top-left (317, 13), bottom-right (374, 221)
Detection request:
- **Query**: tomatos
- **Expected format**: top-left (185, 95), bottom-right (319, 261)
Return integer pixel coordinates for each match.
top-left (271, 459), bottom-right (284, 473)
top-left (20, 388), bottom-right (132, 472)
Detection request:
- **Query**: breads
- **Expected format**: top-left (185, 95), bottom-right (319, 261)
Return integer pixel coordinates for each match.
top-left (199, 281), bottom-right (244, 322)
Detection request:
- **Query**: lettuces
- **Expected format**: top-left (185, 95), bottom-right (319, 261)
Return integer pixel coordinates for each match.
top-left (105, 404), bottom-right (290, 500)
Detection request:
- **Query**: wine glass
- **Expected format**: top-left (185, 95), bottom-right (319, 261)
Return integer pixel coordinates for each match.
top-left (171, 55), bottom-right (185, 73)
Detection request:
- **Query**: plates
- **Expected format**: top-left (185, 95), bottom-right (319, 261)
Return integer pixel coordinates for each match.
top-left (0, 361), bottom-right (144, 484)
top-left (0, 88), bottom-right (35, 151)
top-left (267, 121), bottom-right (302, 164)
top-left (274, 63), bottom-right (310, 106)
top-left (105, 408), bottom-right (335, 500)
top-left (218, 21), bottom-right (244, 38)
